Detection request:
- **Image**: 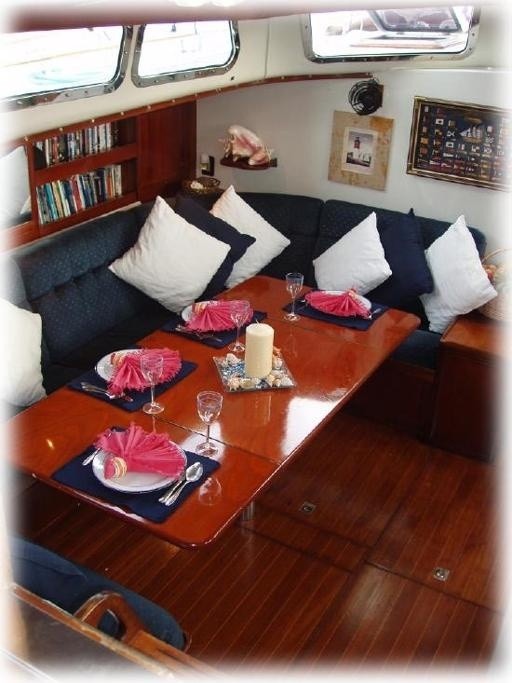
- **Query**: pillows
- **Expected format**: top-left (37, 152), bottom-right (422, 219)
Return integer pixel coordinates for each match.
top-left (205, 184), bottom-right (291, 289)
top-left (159, 179), bottom-right (254, 306)
top-left (417, 214), bottom-right (499, 335)
top-left (107, 196), bottom-right (231, 317)
top-left (376, 208), bottom-right (435, 310)
top-left (309, 210), bottom-right (393, 300)
top-left (0, 295), bottom-right (55, 406)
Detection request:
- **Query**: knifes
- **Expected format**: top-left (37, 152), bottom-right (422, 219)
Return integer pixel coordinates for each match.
top-left (81, 382), bottom-right (133, 403)
top-left (178, 324), bottom-right (223, 343)
top-left (158, 462), bottom-right (201, 503)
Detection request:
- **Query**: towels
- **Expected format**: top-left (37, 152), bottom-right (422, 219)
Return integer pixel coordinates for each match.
top-left (188, 297), bottom-right (250, 332)
top-left (109, 347), bottom-right (182, 394)
top-left (304, 286), bottom-right (369, 317)
top-left (96, 421), bottom-right (185, 477)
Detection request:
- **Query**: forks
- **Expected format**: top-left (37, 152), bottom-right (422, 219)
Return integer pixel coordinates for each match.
top-left (365, 308), bottom-right (381, 321)
top-left (82, 386), bottom-right (118, 400)
top-left (175, 328), bottom-right (213, 340)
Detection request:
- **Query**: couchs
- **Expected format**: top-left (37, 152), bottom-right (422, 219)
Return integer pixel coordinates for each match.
top-left (0, 186), bottom-right (488, 445)
top-left (6, 513), bottom-right (223, 679)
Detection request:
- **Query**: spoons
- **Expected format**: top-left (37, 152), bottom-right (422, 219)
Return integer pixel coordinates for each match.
top-left (165, 461), bottom-right (203, 507)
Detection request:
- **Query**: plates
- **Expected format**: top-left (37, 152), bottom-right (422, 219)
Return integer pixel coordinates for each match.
top-left (181, 300), bottom-right (217, 322)
top-left (305, 290), bottom-right (372, 318)
top-left (91, 441), bottom-right (188, 494)
top-left (95, 348), bottom-right (139, 383)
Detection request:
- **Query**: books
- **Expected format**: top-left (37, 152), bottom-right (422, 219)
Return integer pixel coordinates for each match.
top-left (32, 123), bottom-right (113, 171)
top-left (36, 164), bottom-right (122, 224)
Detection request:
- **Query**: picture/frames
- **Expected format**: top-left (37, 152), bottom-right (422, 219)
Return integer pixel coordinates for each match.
top-left (403, 95), bottom-right (512, 195)
top-left (325, 108), bottom-right (394, 193)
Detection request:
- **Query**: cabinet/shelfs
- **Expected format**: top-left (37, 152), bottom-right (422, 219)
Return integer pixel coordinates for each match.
top-left (2, 102), bottom-right (204, 252)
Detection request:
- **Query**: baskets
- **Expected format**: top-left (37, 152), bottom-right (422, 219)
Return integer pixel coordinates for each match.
top-left (476, 247), bottom-right (512, 323)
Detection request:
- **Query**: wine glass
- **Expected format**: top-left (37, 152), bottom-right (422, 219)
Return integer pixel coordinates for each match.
top-left (284, 272), bottom-right (304, 321)
top-left (141, 354), bottom-right (164, 415)
top-left (227, 302), bottom-right (249, 352)
top-left (195, 390), bottom-right (223, 458)
